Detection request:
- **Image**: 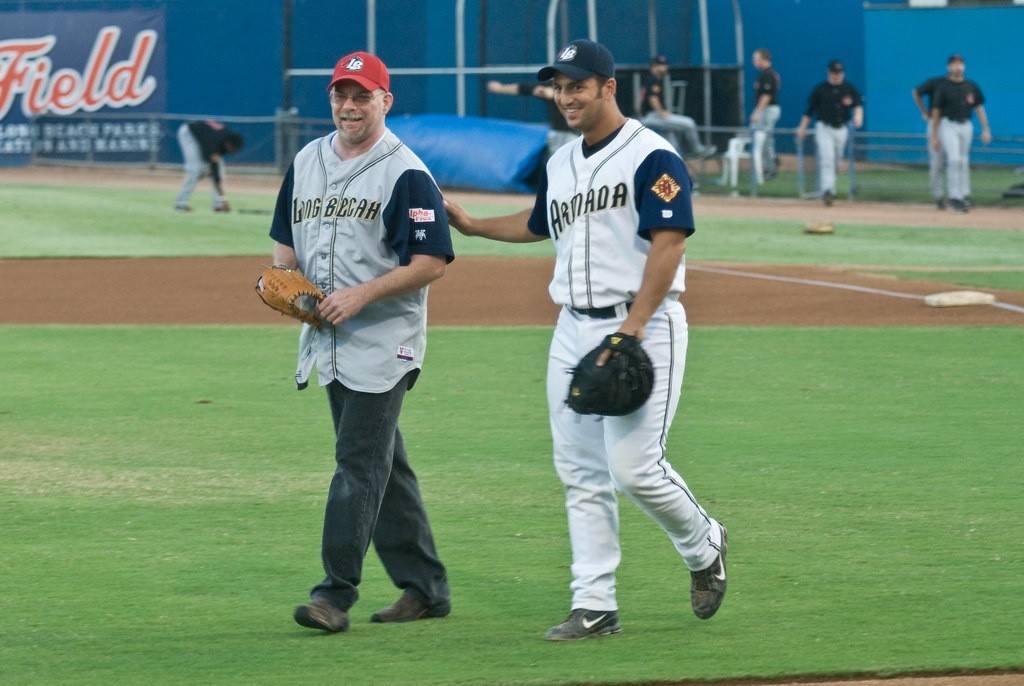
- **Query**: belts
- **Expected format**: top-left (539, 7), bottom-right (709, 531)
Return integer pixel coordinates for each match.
top-left (571, 302), bottom-right (633, 319)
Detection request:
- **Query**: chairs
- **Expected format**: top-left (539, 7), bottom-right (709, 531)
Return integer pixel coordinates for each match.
top-left (722, 136), bottom-right (753, 185)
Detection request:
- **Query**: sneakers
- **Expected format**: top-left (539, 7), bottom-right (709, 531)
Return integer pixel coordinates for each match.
top-left (370, 587), bottom-right (451, 623)
top-left (543, 607), bottom-right (623, 641)
top-left (690, 522), bottom-right (728, 620)
top-left (294, 596), bottom-right (350, 632)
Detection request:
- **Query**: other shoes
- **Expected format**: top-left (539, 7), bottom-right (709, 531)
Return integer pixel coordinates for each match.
top-left (175, 204), bottom-right (194, 213)
top-left (936, 200), bottom-right (946, 211)
top-left (763, 170), bottom-right (776, 180)
top-left (947, 199), bottom-right (969, 214)
top-left (213, 206), bottom-right (231, 213)
top-left (963, 198), bottom-right (976, 209)
top-left (824, 190), bottom-right (833, 206)
top-left (696, 145), bottom-right (719, 160)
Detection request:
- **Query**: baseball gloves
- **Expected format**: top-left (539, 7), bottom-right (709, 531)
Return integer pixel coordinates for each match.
top-left (561, 328), bottom-right (655, 424)
top-left (253, 263), bottom-right (326, 330)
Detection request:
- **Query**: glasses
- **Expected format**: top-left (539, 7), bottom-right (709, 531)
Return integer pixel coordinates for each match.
top-left (329, 90), bottom-right (387, 103)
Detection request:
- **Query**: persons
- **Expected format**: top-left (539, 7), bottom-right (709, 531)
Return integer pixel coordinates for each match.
top-left (796, 60), bottom-right (863, 208)
top-left (640, 55), bottom-right (717, 158)
top-left (255, 51), bottom-right (455, 632)
top-left (487, 80), bottom-right (579, 152)
top-left (751, 50), bottom-right (781, 178)
top-left (914, 75), bottom-right (948, 210)
top-left (932, 53), bottom-right (991, 212)
top-left (442, 40), bottom-right (727, 641)
top-left (173, 118), bottom-right (244, 213)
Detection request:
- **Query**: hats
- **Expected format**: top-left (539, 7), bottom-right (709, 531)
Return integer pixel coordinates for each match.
top-left (537, 39), bottom-right (615, 82)
top-left (650, 55), bottom-right (668, 65)
top-left (830, 60), bottom-right (844, 72)
top-left (948, 54), bottom-right (966, 62)
top-left (325, 52), bottom-right (390, 93)
